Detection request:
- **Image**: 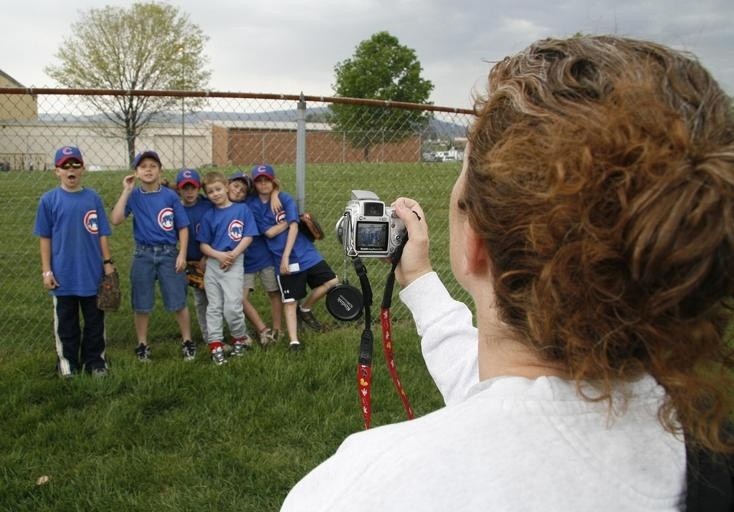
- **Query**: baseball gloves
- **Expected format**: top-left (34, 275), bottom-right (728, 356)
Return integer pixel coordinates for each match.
top-left (299, 213), bottom-right (324, 241)
top-left (184, 265), bottom-right (205, 289)
top-left (96, 268), bottom-right (121, 312)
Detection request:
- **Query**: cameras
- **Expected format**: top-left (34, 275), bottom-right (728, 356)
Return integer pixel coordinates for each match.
top-left (335, 189), bottom-right (406, 258)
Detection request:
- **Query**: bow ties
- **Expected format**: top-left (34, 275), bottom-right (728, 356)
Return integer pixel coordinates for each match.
top-left (91, 369), bottom-right (109, 379)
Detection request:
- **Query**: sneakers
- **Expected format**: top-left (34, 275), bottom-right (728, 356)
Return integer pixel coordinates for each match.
top-left (135, 342), bottom-right (151, 362)
top-left (298, 305), bottom-right (321, 332)
top-left (230, 345), bottom-right (248, 356)
top-left (289, 344), bottom-right (305, 352)
top-left (133, 150), bottom-right (162, 169)
top-left (177, 169), bottom-right (200, 189)
top-left (260, 329), bottom-right (285, 350)
top-left (182, 337), bottom-right (198, 360)
top-left (209, 346), bottom-right (227, 367)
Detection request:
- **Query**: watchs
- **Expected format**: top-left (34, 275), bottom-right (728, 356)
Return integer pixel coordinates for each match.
top-left (104, 259), bottom-right (113, 265)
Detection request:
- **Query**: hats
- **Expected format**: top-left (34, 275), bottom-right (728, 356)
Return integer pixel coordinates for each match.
top-left (252, 165), bottom-right (275, 181)
top-left (54, 146), bottom-right (84, 167)
top-left (228, 172), bottom-right (251, 189)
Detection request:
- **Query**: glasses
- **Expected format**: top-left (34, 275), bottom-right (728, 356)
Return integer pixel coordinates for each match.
top-left (61, 162), bottom-right (82, 169)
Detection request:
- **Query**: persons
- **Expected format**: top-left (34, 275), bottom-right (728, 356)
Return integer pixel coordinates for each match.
top-left (158, 174), bottom-right (282, 348)
top-left (280, 34), bottom-right (731, 512)
top-left (175, 168), bottom-right (252, 353)
top-left (110, 150), bottom-right (196, 365)
top-left (196, 171), bottom-right (260, 367)
top-left (34, 146), bottom-right (115, 380)
top-left (250, 165), bottom-right (339, 352)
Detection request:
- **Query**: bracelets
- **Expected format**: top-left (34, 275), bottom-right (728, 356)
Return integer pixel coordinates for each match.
top-left (42, 270), bottom-right (53, 278)
top-left (162, 177), bottom-right (169, 188)
